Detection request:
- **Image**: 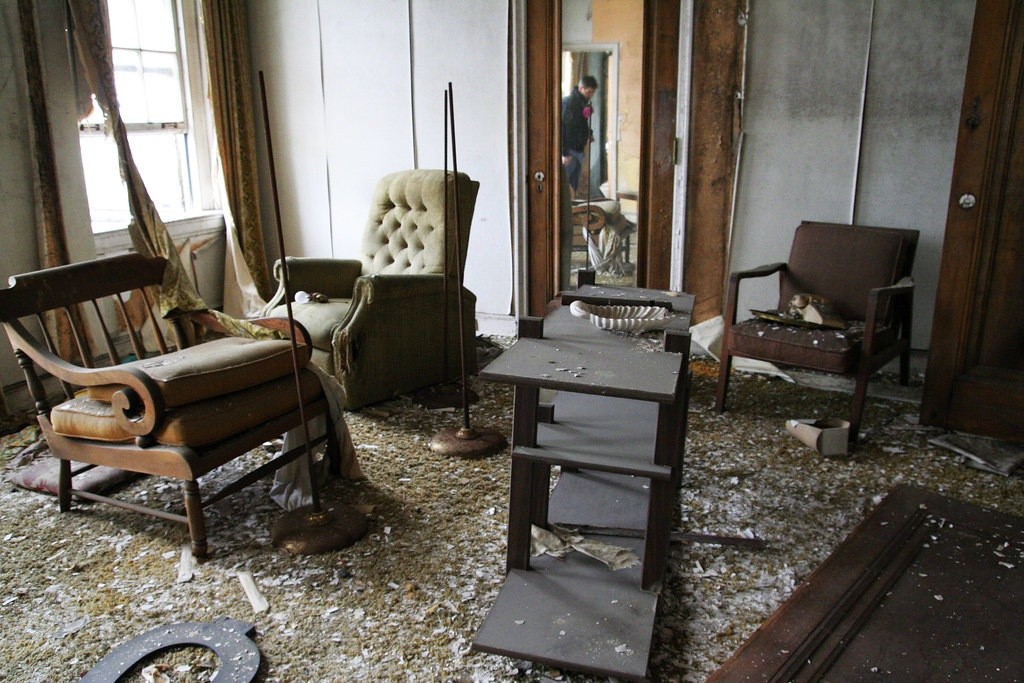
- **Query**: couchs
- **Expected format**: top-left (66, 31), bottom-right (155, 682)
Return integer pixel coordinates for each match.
top-left (259, 165), bottom-right (479, 409)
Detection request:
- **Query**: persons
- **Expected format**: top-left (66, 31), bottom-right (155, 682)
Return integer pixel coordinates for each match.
top-left (561, 75), bottom-right (599, 199)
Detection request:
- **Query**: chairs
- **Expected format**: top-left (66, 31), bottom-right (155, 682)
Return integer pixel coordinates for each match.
top-left (562, 175), bottom-right (635, 265)
top-left (714, 222), bottom-right (919, 448)
top-left (0, 250), bottom-right (342, 557)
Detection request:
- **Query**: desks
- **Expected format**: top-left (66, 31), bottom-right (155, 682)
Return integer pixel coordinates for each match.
top-left (480, 268), bottom-right (697, 591)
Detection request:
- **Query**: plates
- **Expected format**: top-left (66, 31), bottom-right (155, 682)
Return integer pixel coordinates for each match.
top-left (570, 300), bottom-right (674, 332)
